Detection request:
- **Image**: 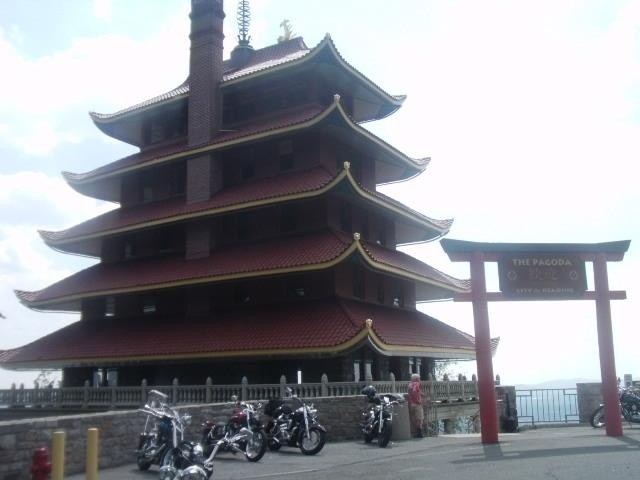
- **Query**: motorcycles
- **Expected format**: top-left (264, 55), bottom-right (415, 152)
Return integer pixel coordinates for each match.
top-left (360, 383), bottom-right (406, 448)
top-left (134, 383), bottom-right (331, 480)
top-left (589, 375), bottom-right (640, 428)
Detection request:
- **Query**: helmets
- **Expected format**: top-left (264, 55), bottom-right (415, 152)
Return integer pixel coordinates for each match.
top-left (362, 385), bottom-right (376, 395)
top-left (173, 440), bottom-right (204, 471)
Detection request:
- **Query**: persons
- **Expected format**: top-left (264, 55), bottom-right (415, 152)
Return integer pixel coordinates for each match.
top-left (406, 373), bottom-right (427, 439)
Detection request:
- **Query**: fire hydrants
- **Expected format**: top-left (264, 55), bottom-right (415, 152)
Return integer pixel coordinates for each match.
top-left (29, 445), bottom-right (53, 480)
